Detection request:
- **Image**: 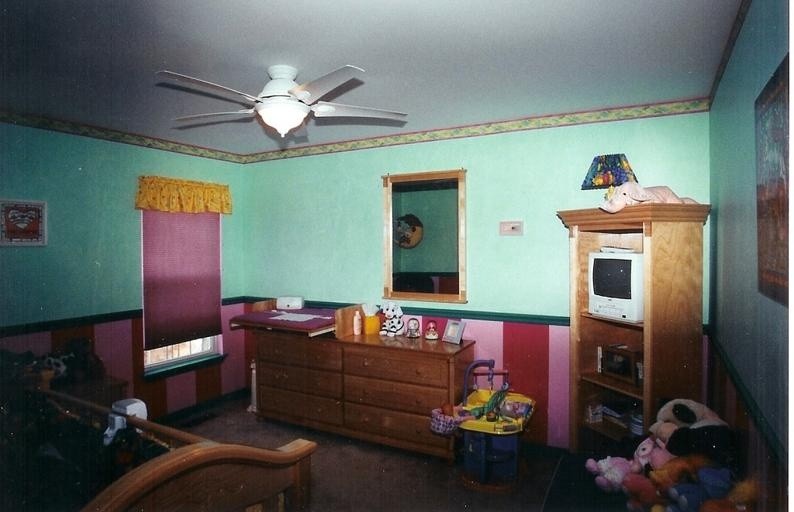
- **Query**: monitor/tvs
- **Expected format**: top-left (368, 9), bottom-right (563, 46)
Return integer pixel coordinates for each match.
top-left (587, 251), bottom-right (644, 324)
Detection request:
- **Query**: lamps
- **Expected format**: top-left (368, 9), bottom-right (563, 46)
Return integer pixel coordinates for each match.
top-left (582, 152), bottom-right (639, 203)
top-left (256, 101), bottom-right (311, 135)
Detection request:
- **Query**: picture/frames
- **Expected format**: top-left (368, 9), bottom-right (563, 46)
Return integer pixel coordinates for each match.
top-left (1, 200), bottom-right (46, 246)
top-left (442, 319), bottom-right (465, 344)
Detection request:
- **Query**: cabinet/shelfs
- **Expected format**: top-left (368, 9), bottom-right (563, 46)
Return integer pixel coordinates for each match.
top-left (557, 205), bottom-right (713, 454)
top-left (252, 330), bottom-right (476, 468)
top-left (44, 376), bottom-right (128, 409)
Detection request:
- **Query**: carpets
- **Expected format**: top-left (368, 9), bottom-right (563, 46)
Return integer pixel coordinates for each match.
top-left (540, 457), bottom-right (628, 512)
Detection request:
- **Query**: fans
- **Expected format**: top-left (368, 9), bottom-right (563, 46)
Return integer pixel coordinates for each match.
top-left (156, 66), bottom-right (408, 135)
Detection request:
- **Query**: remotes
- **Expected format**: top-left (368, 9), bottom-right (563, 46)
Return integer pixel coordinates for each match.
top-left (600, 245), bottom-right (634, 254)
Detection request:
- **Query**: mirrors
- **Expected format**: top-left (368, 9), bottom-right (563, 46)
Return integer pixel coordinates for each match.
top-left (382, 169), bottom-right (466, 306)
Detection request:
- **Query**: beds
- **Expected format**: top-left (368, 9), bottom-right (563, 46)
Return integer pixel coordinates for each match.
top-left (0, 373), bottom-right (319, 512)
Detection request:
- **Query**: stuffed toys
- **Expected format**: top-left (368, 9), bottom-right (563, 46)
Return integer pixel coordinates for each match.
top-left (599, 180), bottom-right (700, 214)
top-left (378, 301), bottom-right (405, 337)
top-left (583, 394), bottom-right (762, 512)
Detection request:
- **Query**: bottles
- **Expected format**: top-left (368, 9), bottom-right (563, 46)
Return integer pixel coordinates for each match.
top-left (352, 309), bottom-right (362, 337)
